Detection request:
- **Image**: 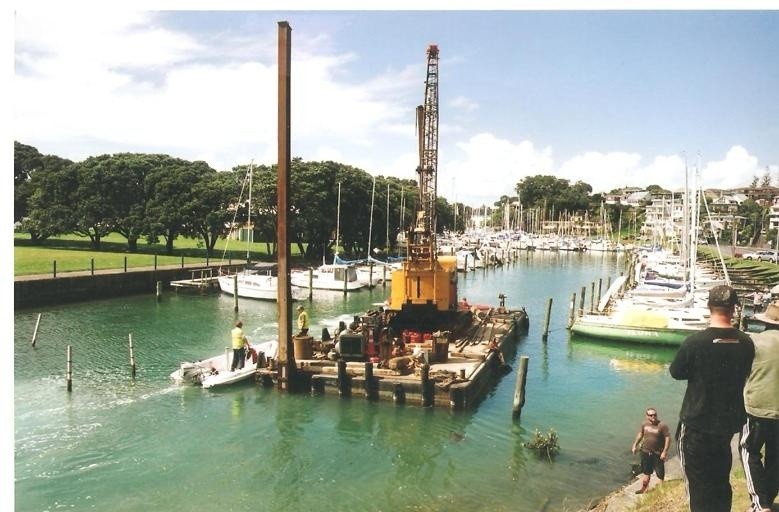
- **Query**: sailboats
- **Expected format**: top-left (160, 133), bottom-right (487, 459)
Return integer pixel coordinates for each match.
top-left (290, 182), bottom-right (365, 291)
top-left (330, 149), bottom-right (748, 349)
top-left (216, 158), bottom-right (313, 301)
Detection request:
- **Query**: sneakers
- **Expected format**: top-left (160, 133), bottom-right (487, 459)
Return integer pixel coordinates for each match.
top-left (635, 489), bottom-right (644, 494)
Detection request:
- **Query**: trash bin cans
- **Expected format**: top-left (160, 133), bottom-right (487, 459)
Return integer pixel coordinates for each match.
top-left (431, 336), bottom-right (449, 363)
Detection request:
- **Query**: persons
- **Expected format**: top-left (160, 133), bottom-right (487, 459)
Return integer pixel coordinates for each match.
top-left (667, 283), bottom-right (756, 512)
top-left (737, 301), bottom-right (779, 512)
top-left (760, 289), bottom-right (772, 312)
top-left (748, 287), bottom-right (764, 314)
top-left (229, 320), bottom-right (251, 373)
top-left (295, 305), bottom-right (309, 338)
top-left (630, 406), bottom-right (673, 495)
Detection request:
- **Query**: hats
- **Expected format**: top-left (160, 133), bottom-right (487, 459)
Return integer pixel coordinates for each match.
top-left (707, 285), bottom-right (744, 309)
top-left (752, 299), bottom-right (779, 324)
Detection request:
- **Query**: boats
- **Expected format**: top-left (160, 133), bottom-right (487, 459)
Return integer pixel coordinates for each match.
top-left (169, 336), bottom-right (284, 395)
